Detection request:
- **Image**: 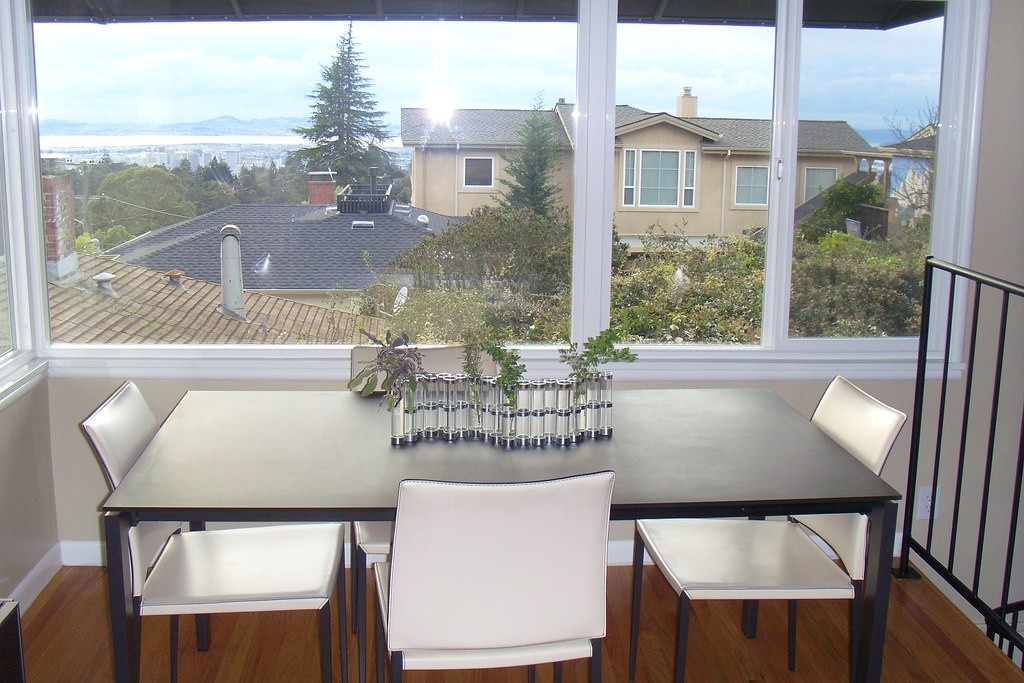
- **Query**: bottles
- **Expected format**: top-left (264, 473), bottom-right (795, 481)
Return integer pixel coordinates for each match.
top-left (391, 373), bottom-right (612, 446)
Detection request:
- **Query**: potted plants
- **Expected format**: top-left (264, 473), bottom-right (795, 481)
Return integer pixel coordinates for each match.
top-left (346, 325), bottom-right (641, 448)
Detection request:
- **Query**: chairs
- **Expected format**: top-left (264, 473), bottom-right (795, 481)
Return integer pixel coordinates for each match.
top-left (78, 340), bottom-right (911, 677)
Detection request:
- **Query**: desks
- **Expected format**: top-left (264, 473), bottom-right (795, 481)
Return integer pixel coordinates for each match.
top-left (100, 390), bottom-right (903, 683)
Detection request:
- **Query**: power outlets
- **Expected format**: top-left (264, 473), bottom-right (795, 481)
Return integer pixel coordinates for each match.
top-left (916, 487), bottom-right (942, 520)
